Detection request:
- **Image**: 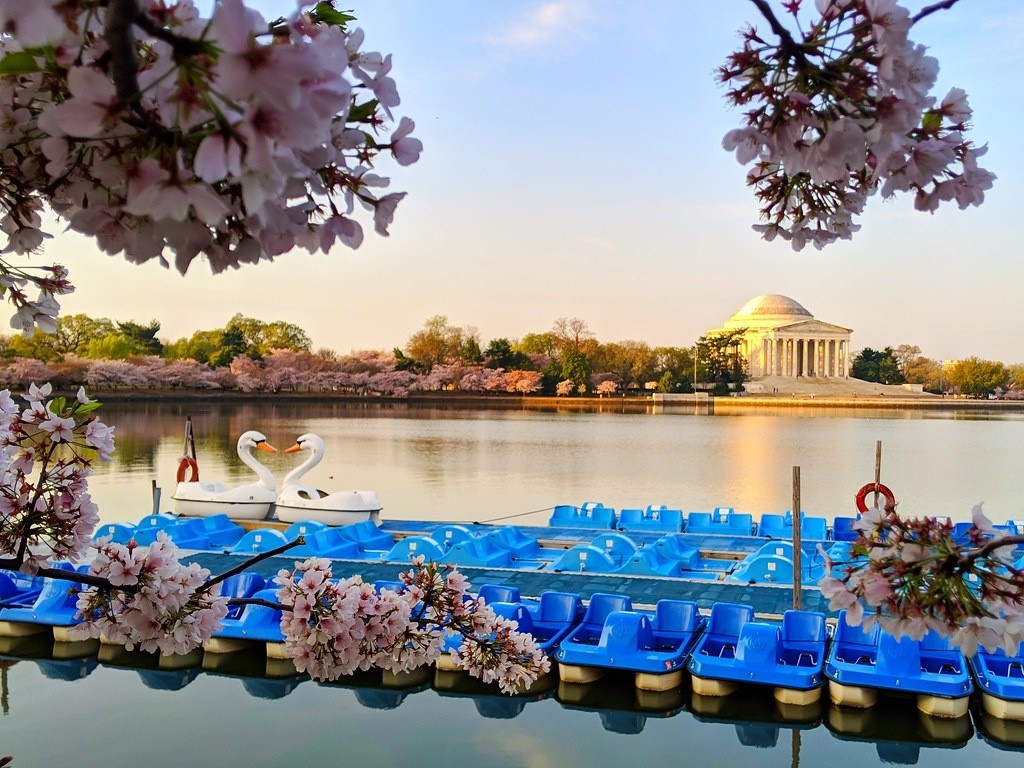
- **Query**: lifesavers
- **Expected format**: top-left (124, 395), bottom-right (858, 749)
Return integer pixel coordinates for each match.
top-left (176, 457), bottom-right (199, 483)
top-left (855, 482), bottom-right (896, 518)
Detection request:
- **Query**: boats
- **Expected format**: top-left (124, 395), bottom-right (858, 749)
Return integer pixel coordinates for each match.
top-left (274, 434), bottom-right (384, 528)
top-left (1, 501), bottom-right (1024, 722)
top-left (171, 431), bottom-right (277, 520)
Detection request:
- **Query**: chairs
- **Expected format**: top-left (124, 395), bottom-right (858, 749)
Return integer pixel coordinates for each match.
top-left (0, 489), bottom-right (1024, 674)
